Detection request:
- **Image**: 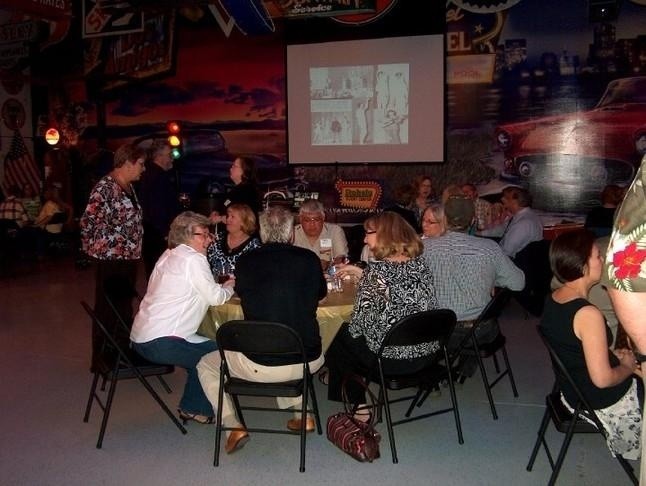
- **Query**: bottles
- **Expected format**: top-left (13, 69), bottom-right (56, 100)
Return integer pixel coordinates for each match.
top-left (293, 191), bottom-right (319, 206)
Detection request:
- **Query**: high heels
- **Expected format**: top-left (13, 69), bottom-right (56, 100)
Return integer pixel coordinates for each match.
top-left (177, 408), bottom-right (216, 427)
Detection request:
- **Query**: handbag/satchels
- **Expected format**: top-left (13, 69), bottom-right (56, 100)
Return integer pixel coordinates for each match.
top-left (325, 408), bottom-right (383, 464)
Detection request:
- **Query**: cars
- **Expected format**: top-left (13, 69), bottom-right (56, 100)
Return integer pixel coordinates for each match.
top-left (56, 117), bottom-right (297, 228)
top-left (491, 68), bottom-right (645, 213)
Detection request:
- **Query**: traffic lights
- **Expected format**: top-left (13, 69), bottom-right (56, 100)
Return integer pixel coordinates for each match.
top-left (165, 118), bottom-right (184, 162)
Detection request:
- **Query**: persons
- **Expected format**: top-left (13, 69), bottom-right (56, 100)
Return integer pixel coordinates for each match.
top-left (35, 185), bottom-right (65, 235)
top-left (69, 126), bottom-right (114, 266)
top-left (311, 70), bottom-right (409, 143)
top-left (130, 137), bottom-right (544, 454)
top-left (1, 185), bottom-right (32, 240)
top-left (65, 181), bottom-right (88, 236)
top-left (539, 148), bottom-right (646, 485)
top-left (78, 142), bottom-right (147, 372)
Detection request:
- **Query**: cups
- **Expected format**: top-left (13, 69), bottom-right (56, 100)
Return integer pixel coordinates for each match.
top-left (215, 267), bottom-right (230, 285)
top-left (180, 193), bottom-right (189, 208)
top-left (332, 273), bottom-right (350, 293)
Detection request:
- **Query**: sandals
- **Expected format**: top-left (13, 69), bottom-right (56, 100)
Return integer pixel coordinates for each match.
top-left (319, 369), bottom-right (329, 385)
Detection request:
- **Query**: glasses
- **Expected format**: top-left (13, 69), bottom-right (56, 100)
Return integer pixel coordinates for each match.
top-left (422, 215), bottom-right (440, 225)
top-left (192, 231), bottom-right (211, 240)
top-left (362, 230), bottom-right (376, 239)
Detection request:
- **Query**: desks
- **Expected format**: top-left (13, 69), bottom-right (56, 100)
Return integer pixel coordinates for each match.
top-left (196, 282), bottom-right (358, 357)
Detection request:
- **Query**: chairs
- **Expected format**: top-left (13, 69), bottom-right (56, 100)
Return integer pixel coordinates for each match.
top-left (417, 288), bottom-right (519, 419)
top-left (212, 320), bottom-right (323, 472)
top-left (0, 210), bottom-right (70, 278)
top-left (509, 239), bottom-right (553, 322)
top-left (80, 300), bottom-right (187, 449)
top-left (95, 275), bottom-right (173, 394)
top-left (526, 325), bottom-right (639, 486)
top-left (352, 308), bottom-right (464, 464)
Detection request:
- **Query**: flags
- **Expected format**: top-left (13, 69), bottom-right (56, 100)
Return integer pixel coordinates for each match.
top-left (0, 129), bottom-right (40, 200)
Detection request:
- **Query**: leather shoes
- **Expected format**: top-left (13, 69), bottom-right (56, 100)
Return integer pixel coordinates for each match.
top-left (223, 425), bottom-right (250, 454)
top-left (287, 416), bottom-right (315, 433)
top-left (352, 407), bottom-right (373, 423)
